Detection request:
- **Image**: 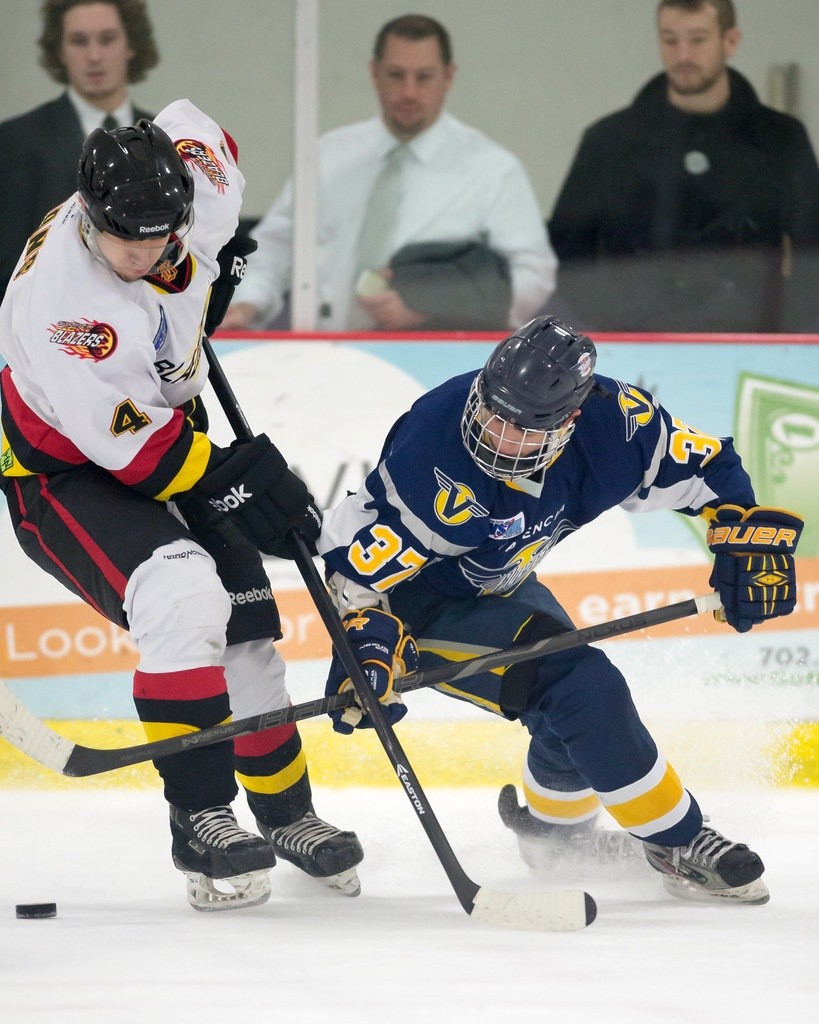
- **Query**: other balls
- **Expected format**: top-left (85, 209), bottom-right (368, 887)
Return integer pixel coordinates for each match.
top-left (16, 901), bottom-right (58, 919)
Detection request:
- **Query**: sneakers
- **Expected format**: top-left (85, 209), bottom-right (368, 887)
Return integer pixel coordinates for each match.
top-left (500, 784), bottom-right (646, 899)
top-left (257, 806), bottom-right (364, 898)
top-left (643, 824), bottom-right (770, 905)
top-left (169, 802), bottom-right (276, 913)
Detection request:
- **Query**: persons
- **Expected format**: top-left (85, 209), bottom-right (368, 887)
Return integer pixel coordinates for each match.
top-left (217, 13), bottom-right (559, 332)
top-left (0, 90), bottom-right (366, 910)
top-left (540, 0), bottom-right (819, 335)
top-left (314, 312), bottom-right (807, 905)
top-left (0, 1), bottom-right (162, 305)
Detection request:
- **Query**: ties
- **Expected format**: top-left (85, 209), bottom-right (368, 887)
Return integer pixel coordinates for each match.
top-left (345, 144), bottom-right (419, 337)
top-left (104, 117), bottom-right (115, 128)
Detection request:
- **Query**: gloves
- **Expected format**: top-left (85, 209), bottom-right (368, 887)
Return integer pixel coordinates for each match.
top-left (706, 504), bottom-right (804, 634)
top-left (324, 609), bottom-right (419, 737)
top-left (166, 433), bottom-right (323, 563)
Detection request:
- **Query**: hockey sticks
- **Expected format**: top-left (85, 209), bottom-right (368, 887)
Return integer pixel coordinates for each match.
top-left (195, 330), bottom-right (602, 934)
top-left (0, 581), bottom-right (784, 784)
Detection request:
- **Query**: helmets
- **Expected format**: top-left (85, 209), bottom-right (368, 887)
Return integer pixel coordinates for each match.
top-left (76, 120), bottom-right (196, 240)
top-left (463, 315), bottom-right (598, 476)
